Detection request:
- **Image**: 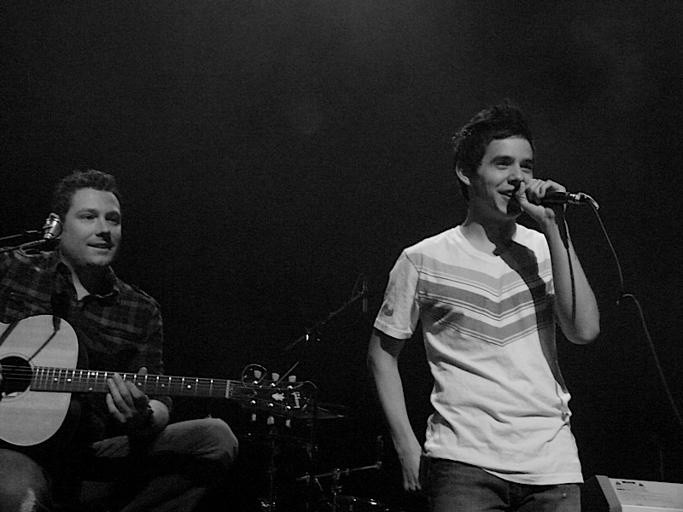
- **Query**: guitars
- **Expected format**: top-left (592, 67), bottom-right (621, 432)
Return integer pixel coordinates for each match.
top-left (1, 314), bottom-right (315, 447)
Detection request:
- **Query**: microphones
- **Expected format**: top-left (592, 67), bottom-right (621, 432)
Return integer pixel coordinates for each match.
top-left (43, 212), bottom-right (62, 241)
top-left (525, 187), bottom-right (587, 207)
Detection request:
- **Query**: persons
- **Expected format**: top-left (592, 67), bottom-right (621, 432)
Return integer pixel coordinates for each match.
top-left (364, 97), bottom-right (604, 512)
top-left (0, 168), bottom-right (240, 511)
